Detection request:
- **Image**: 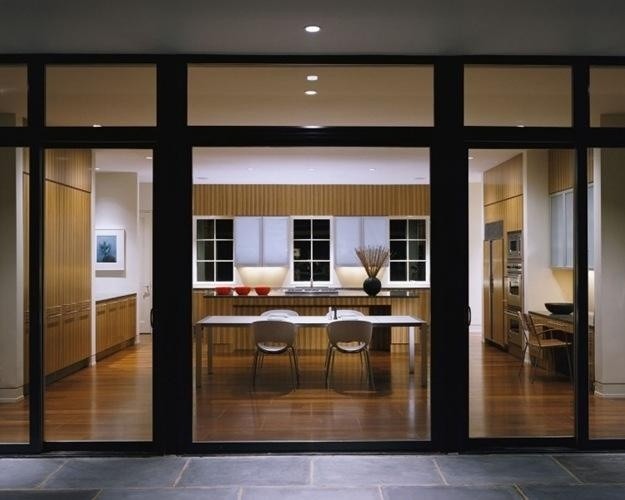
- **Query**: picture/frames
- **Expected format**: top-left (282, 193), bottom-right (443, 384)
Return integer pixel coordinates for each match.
top-left (95, 228), bottom-right (125, 271)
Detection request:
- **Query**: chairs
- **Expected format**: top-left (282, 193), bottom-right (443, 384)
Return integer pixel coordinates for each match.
top-left (516, 311), bottom-right (574, 384)
top-left (252, 309), bottom-right (377, 393)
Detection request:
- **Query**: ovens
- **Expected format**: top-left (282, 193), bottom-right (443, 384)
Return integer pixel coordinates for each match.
top-left (503, 309), bottom-right (523, 349)
top-left (503, 231), bottom-right (524, 268)
top-left (505, 271), bottom-right (523, 311)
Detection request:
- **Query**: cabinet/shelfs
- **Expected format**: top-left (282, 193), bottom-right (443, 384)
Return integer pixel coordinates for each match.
top-left (22, 120), bottom-right (92, 396)
top-left (96, 293), bottom-right (136, 362)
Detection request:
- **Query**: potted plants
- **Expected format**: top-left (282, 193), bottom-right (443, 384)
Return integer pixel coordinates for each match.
top-left (355, 245), bottom-right (391, 296)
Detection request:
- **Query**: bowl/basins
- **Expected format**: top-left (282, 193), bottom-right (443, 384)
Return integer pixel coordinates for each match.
top-left (254, 286), bottom-right (271, 296)
top-left (234, 287), bottom-right (251, 295)
top-left (544, 302), bottom-right (574, 315)
top-left (213, 287), bottom-right (232, 296)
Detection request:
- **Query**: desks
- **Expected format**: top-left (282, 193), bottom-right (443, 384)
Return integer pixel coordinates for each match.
top-left (194, 316), bottom-right (427, 387)
top-left (530, 309), bottom-right (595, 392)
